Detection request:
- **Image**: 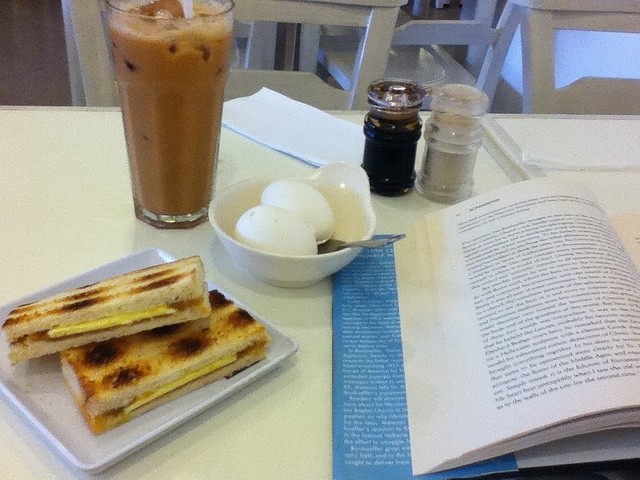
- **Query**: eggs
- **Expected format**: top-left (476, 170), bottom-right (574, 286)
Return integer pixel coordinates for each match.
top-left (235, 204), bottom-right (319, 257)
top-left (259, 180), bottom-right (335, 241)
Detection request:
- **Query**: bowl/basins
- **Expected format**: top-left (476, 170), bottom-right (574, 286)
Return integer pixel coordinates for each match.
top-left (208, 179), bottom-right (377, 289)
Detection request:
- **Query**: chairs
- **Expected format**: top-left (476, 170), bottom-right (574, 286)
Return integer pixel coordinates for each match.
top-left (318, 0), bottom-right (518, 113)
top-left (517, 2), bottom-right (639, 116)
top-left (69, 1), bottom-right (407, 111)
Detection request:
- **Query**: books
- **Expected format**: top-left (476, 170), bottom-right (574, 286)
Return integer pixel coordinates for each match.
top-left (331, 170), bottom-right (639, 479)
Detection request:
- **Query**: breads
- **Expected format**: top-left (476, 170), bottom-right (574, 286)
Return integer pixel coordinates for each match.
top-left (60, 289), bottom-right (273, 435)
top-left (2, 255), bottom-right (211, 367)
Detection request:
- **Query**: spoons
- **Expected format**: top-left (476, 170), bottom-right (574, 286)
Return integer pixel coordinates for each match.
top-left (317, 234), bottom-right (406, 252)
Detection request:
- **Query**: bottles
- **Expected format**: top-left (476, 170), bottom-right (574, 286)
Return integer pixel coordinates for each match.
top-left (415, 84), bottom-right (490, 205)
top-left (362, 77), bottom-right (427, 197)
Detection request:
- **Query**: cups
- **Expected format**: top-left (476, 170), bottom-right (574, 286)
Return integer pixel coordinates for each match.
top-left (99, 0), bottom-right (234, 229)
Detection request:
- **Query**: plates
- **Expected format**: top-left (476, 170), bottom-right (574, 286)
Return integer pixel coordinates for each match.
top-left (1, 247), bottom-right (298, 474)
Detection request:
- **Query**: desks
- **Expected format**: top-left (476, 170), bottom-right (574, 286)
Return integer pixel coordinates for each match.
top-left (0, 107), bottom-right (640, 480)
